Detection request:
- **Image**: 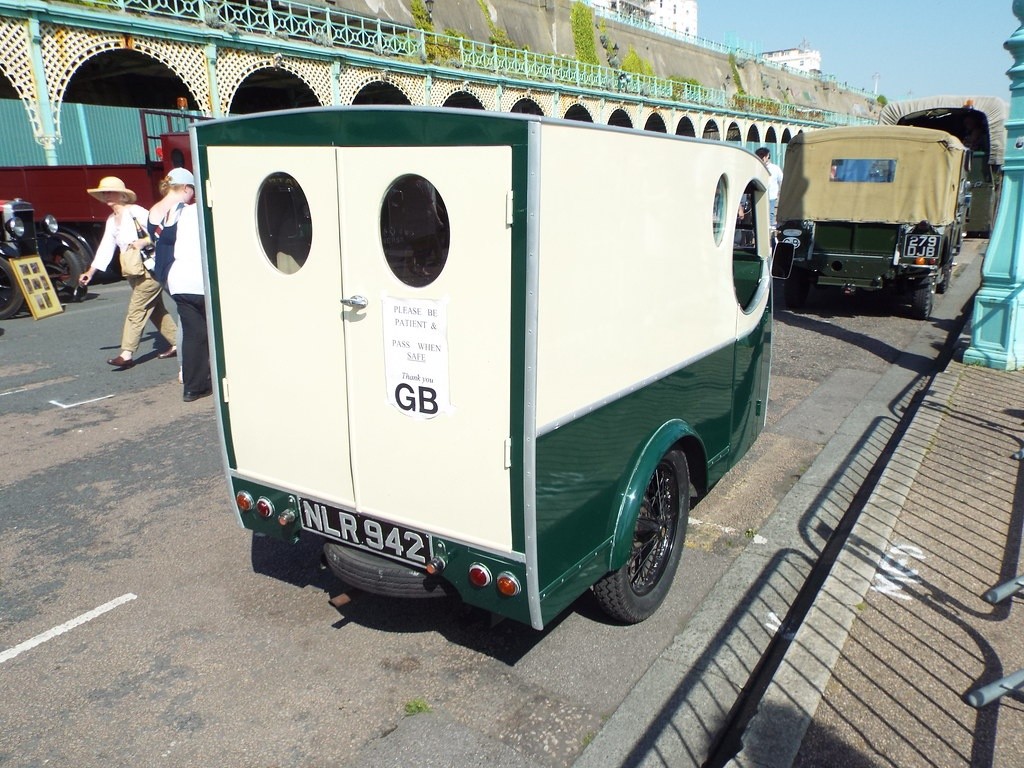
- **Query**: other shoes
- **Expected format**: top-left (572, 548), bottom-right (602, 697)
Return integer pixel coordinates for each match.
top-left (178, 372), bottom-right (184, 384)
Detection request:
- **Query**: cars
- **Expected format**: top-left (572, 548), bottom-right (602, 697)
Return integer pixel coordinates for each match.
top-left (0, 194), bottom-right (96, 322)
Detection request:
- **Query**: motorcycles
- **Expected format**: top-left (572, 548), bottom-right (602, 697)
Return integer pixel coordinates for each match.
top-left (188, 104), bottom-right (797, 635)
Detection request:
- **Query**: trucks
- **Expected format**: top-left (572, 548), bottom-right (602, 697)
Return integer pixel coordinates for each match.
top-left (877, 94), bottom-right (1010, 240)
top-left (773, 122), bottom-right (974, 321)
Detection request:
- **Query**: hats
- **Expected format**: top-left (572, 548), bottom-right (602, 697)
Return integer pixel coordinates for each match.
top-left (167, 167), bottom-right (195, 186)
top-left (87, 176), bottom-right (137, 203)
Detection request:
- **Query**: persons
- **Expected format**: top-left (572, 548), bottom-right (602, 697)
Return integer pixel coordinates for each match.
top-left (734, 148), bottom-right (784, 257)
top-left (167, 201), bottom-right (213, 401)
top-left (79, 177), bottom-right (176, 368)
top-left (147, 167), bottom-right (195, 384)
top-left (300, 199), bottom-right (311, 246)
top-left (401, 175), bottom-right (448, 277)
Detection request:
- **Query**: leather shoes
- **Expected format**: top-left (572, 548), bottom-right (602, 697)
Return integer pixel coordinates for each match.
top-left (107, 356), bottom-right (132, 366)
top-left (159, 348), bottom-right (178, 359)
top-left (184, 388), bottom-right (212, 401)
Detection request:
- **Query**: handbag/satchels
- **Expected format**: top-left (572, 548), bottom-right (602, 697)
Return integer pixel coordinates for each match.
top-left (120, 243), bottom-right (144, 277)
